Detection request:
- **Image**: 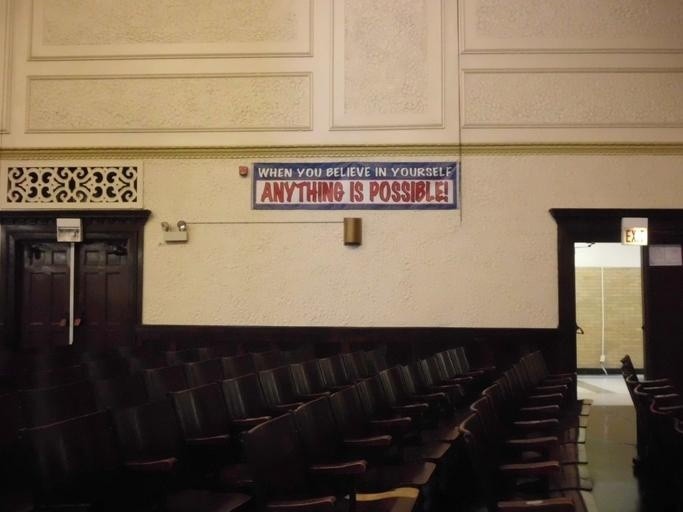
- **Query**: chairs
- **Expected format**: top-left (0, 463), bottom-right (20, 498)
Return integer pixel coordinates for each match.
top-left (2, 345), bottom-right (588, 510)
top-left (620, 353), bottom-right (682, 511)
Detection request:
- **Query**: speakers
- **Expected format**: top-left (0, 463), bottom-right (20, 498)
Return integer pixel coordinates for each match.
top-left (344, 218), bottom-right (361, 246)
